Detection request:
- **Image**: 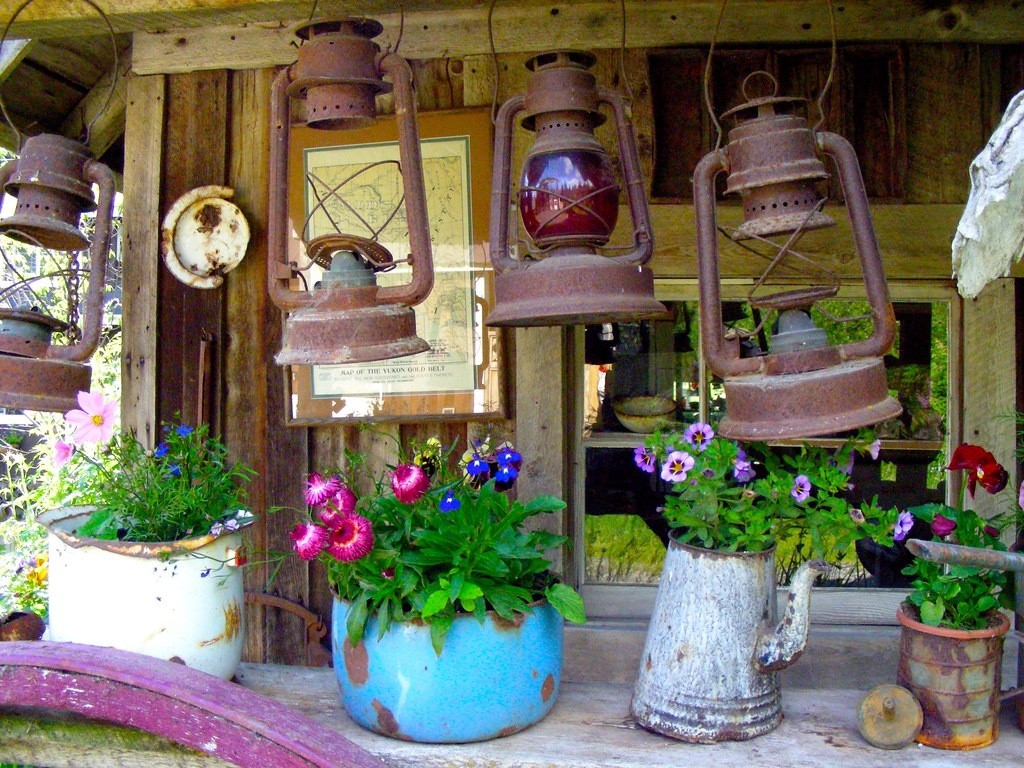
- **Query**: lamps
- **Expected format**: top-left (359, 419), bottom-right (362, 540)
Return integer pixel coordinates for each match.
top-left (0, 0), bottom-right (117, 413)
top-left (483, 0), bottom-right (672, 325)
top-left (694, 0), bottom-right (900, 441)
top-left (266, 0), bottom-right (434, 366)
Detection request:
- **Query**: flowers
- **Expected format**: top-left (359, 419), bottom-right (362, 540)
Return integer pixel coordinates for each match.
top-left (164, 414), bottom-right (589, 660)
top-left (632, 421), bottom-right (915, 567)
top-left (40, 388), bottom-right (250, 546)
top-left (899, 440), bottom-right (1024, 629)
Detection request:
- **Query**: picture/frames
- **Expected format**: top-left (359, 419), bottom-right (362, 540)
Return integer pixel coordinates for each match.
top-left (282, 108), bottom-right (508, 427)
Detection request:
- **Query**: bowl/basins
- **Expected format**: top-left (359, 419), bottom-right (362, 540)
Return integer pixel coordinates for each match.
top-left (614, 396), bottom-right (678, 432)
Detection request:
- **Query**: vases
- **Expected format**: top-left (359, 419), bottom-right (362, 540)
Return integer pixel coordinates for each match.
top-left (895, 604), bottom-right (1011, 751)
top-left (45, 507), bottom-right (254, 681)
top-left (631, 530), bottom-right (834, 745)
top-left (331, 569), bottom-right (569, 746)
